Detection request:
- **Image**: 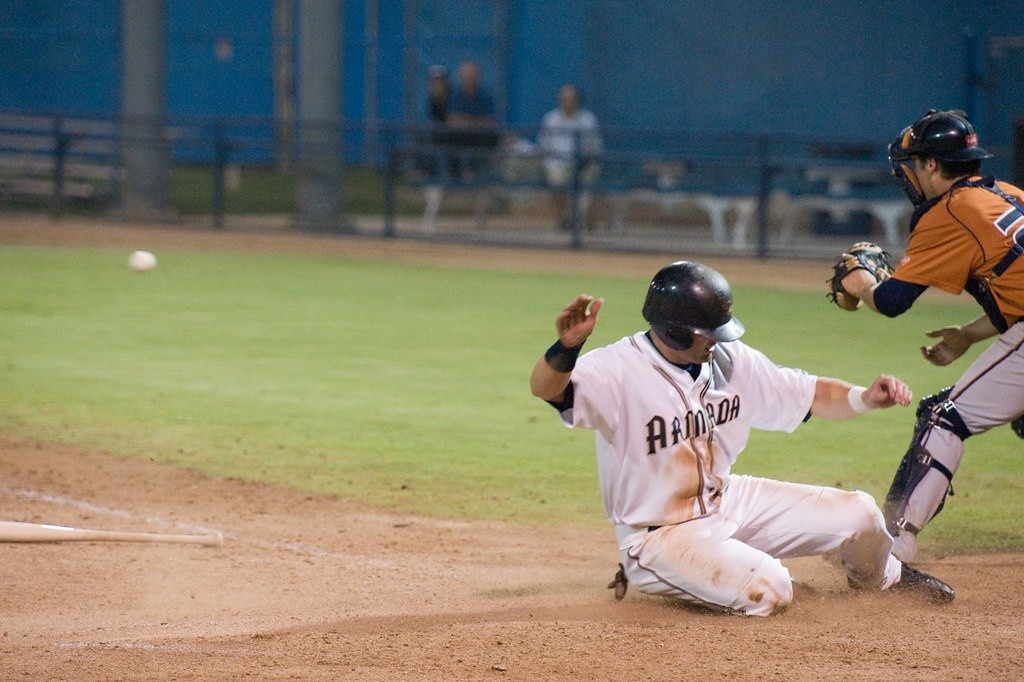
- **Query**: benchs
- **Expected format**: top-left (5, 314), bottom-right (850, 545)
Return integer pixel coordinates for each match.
top-left (421, 161), bottom-right (913, 246)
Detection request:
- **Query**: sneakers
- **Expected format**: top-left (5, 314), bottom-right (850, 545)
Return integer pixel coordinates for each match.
top-left (846, 560), bottom-right (956, 606)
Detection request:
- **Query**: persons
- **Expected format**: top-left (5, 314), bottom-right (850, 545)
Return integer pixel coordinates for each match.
top-left (834, 105), bottom-right (1024, 563)
top-left (530, 259), bottom-right (960, 617)
top-left (537, 83), bottom-right (602, 250)
top-left (422, 58), bottom-right (503, 239)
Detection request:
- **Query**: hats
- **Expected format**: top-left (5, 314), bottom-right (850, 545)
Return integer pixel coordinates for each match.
top-left (426, 64), bottom-right (448, 79)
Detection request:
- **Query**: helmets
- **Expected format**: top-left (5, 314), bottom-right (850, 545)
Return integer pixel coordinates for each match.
top-left (641, 260), bottom-right (746, 351)
top-left (908, 107), bottom-right (994, 163)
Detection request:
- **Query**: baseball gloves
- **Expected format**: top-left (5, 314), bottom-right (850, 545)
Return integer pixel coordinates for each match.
top-left (830, 238), bottom-right (884, 315)
